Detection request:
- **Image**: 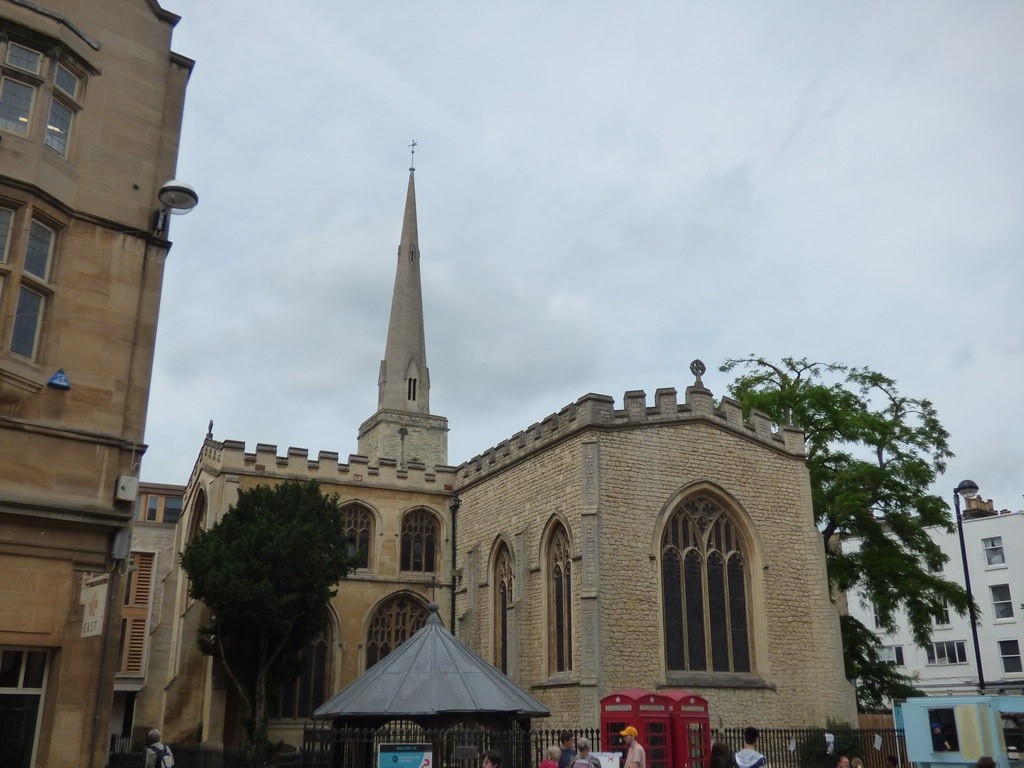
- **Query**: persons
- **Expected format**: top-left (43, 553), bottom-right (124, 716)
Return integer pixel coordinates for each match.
top-left (976, 757), bottom-right (996, 768)
top-left (931, 723), bottom-right (951, 751)
top-left (837, 756), bottom-right (863, 768)
top-left (886, 755), bottom-right (898, 768)
top-left (620, 726), bottom-right (646, 768)
top-left (735, 727), bottom-right (766, 768)
top-left (145, 729), bottom-right (174, 768)
top-left (710, 741), bottom-right (731, 768)
top-left (540, 746), bottom-right (561, 768)
top-left (558, 732), bottom-right (576, 768)
top-left (570, 738), bottom-right (602, 768)
top-left (481, 750), bottom-right (502, 768)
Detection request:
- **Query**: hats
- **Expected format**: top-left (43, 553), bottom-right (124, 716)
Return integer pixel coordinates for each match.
top-left (148, 729), bottom-right (161, 741)
top-left (620, 727), bottom-right (638, 737)
top-left (931, 722), bottom-right (943, 728)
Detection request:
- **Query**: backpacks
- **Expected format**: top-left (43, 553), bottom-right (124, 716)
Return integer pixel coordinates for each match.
top-left (570, 755), bottom-right (594, 768)
top-left (151, 745), bottom-right (175, 768)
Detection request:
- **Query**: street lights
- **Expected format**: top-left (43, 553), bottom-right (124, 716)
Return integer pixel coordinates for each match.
top-left (954, 480), bottom-right (986, 695)
